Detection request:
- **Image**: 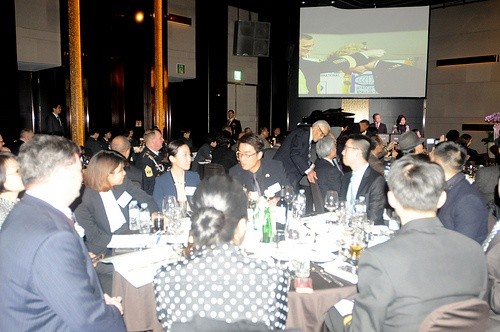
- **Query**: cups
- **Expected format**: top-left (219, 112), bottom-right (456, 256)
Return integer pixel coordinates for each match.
top-left (324, 190), bottom-right (375, 260)
top-left (284, 185), bottom-right (295, 205)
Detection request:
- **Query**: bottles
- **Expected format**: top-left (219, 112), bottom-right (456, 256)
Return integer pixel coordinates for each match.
top-left (298, 188), bottom-right (305, 214)
top-left (356, 195), bottom-right (366, 215)
top-left (262, 196), bottom-right (272, 237)
top-left (276, 185), bottom-right (287, 228)
top-left (153, 195), bottom-right (186, 232)
top-left (139, 202), bottom-right (148, 232)
top-left (129, 200), bottom-right (139, 231)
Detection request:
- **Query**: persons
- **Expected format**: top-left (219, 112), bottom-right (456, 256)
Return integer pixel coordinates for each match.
top-left (398, 131), bottom-right (426, 154)
top-left (331, 113), bottom-right (421, 146)
top-left (0, 127), bottom-right (128, 332)
top-left (46, 104), bottom-right (64, 137)
top-left (272, 120), bottom-right (331, 191)
top-left (85, 127), bottom-right (165, 196)
top-left (367, 134), bottom-right (384, 175)
top-left (224, 110), bottom-right (242, 137)
top-left (435, 130), bottom-right (479, 165)
top-left (74, 148), bottom-right (159, 297)
top-left (341, 134), bottom-right (385, 226)
top-left (324, 153), bottom-right (489, 332)
top-left (228, 133), bottom-right (288, 207)
top-left (259, 126), bottom-right (280, 148)
top-left (299, 33), bottom-right (384, 94)
top-left (335, 43), bottom-right (425, 94)
top-left (313, 135), bottom-right (343, 199)
top-left (485, 239), bottom-right (500, 332)
top-left (179, 128), bottom-right (238, 180)
top-left (152, 172), bottom-right (288, 332)
top-left (152, 138), bottom-right (201, 211)
top-left (431, 141), bottom-right (489, 245)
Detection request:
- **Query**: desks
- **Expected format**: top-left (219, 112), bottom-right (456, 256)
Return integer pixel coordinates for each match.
top-left (112, 210), bottom-right (400, 332)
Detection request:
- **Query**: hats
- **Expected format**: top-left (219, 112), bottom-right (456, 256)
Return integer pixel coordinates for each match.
top-left (396, 130), bottom-right (426, 150)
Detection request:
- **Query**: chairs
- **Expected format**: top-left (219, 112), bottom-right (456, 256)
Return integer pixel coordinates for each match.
top-left (419, 298), bottom-right (491, 332)
top-left (310, 178), bottom-right (326, 213)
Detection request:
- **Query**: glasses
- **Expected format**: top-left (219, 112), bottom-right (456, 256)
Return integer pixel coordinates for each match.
top-left (236, 150), bottom-right (258, 159)
top-left (317, 126), bottom-right (326, 138)
